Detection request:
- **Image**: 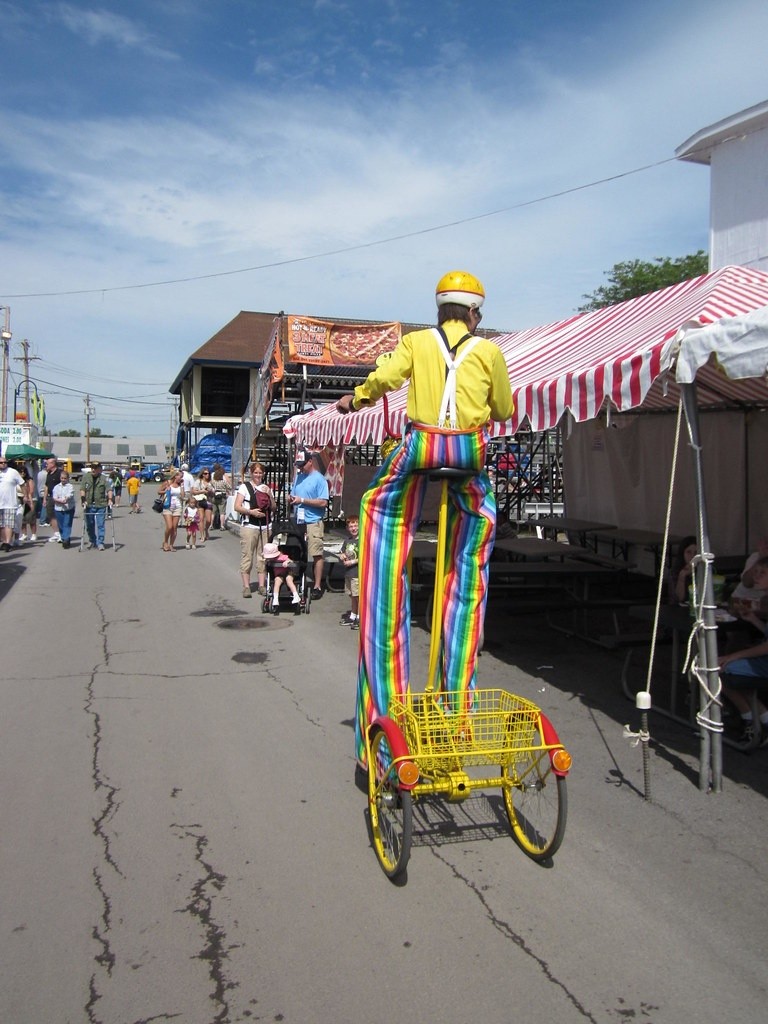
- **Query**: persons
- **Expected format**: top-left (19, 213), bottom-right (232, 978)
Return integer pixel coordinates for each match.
top-left (717, 556), bottom-right (768, 742)
top-left (287, 450), bottom-right (329, 601)
top-left (261, 542), bottom-right (301, 606)
top-left (338, 516), bottom-right (360, 630)
top-left (53, 471), bottom-right (76, 549)
top-left (335, 271), bottom-right (516, 643)
top-left (157, 463), bottom-right (232, 552)
top-left (79, 461), bottom-right (114, 551)
top-left (108, 468), bottom-right (141, 514)
top-left (669, 535), bottom-right (697, 607)
top-left (234, 462), bottom-right (276, 599)
top-left (496, 511), bottom-right (518, 539)
top-left (0, 457), bottom-right (64, 553)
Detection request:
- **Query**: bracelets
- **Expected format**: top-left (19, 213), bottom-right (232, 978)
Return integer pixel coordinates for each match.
top-left (248, 510), bottom-right (250, 515)
top-left (301, 498), bottom-right (304, 503)
top-left (352, 560), bottom-right (355, 564)
top-left (107, 498), bottom-right (112, 500)
top-left (81, 496), bottom-right (85, 503)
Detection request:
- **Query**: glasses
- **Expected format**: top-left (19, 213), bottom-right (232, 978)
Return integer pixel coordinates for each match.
top-left (20, 471), bottom-right (26, 473)
top-left (203, 473), bottom-right (208, 475)
top-left (0, 462), bottom-right (7, 464)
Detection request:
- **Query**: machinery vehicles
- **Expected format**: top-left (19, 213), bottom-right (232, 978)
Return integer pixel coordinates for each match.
top-left (135, 463), bottom-right (166, 483)
top-left (127, 454), bottom-right (146, 473)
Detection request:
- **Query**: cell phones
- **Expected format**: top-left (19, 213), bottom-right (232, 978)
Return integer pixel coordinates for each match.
top-left (289, 495), bottom-right (295, 501)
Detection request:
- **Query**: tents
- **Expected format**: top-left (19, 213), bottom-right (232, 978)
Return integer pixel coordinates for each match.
top-left (0, 440), bottom-right (55, 460)
top-left (284, 265), bottom-right (768, 793)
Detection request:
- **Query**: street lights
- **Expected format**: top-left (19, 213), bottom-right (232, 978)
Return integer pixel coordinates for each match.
top-left (13, 380), bottom-right (41, 422)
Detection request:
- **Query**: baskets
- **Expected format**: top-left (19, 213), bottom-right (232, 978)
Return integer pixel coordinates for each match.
top-left (391, 687), bottom-right (541, 772)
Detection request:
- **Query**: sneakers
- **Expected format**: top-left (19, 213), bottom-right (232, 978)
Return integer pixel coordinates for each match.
top-left (242, 587), bottom-right (252, 598)
top-left (352, 618), bottom-right (360, 630)
top-left (258, 586), bottom-right (267, 596)
top-left (340, 614), bottom-right (357, 626)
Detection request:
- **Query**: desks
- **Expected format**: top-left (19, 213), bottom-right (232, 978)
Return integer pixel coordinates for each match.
top-left (526, 516), bottom-right (618, 548)
top-left (709, 553), bottom-right (751, 590)
top-left (422, 561), bottom-right (615, 638)
top-left (627, 601), bottom-right (768, 722)
top-left (586, 526), bottom-right (684, 595)
top-left (488, 535), bottom-right (589, 600)
top-left (421, 526), bottom-right (438, 536)
top-left (409, 541), bottom-right (438, 598)
top-left (329, 528), bottom-right (355, 592)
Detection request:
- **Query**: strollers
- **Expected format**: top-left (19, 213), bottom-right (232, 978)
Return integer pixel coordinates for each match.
top-left (262, 516), bottom-right (313, 614)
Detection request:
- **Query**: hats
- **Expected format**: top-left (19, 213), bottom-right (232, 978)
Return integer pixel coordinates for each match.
top-left (91, 461), bottom-right (102, 468)
top-left (130, 471), bottom-right (135, 477)
top-left (262, 543), bottom-right (280, 558)
top-left (294, 450), bottom-right (313, 467)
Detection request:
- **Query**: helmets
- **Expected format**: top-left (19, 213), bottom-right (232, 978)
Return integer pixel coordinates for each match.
top-left (436, 271), bottom-right (486, 310)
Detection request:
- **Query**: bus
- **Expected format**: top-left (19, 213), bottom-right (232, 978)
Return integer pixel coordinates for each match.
top-left (100, 463), bottom-right (131, 479)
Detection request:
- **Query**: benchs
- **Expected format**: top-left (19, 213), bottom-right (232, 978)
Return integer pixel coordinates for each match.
top-left (321, 543), bottom-right (768, 752)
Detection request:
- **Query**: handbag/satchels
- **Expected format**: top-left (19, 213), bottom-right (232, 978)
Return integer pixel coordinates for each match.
top-left (164, 488), bottom-right (171, 508)
top-left (214, 490), bottom-right (224, 499)
top-left (114, 477), bottom-right (122, 488)
top-left (152, 493), bottom-right (163, 513)
top-left (199, 499), bottom-right (208, 509)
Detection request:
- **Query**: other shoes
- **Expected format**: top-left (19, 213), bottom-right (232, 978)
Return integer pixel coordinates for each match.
top-left (292, 595), bottom-right (301, 604)
top-left (310, 586), bottom-right (325, 599)
top-left (0, 520), bottom-right (106, 551)
top-left (160, 526), bottom-right (226, 552)
top-left (273, 598), bottom-right (279, 606)
top-left (137, 508), bottom-right (141, 513)
top-left (129, 511), bottom-right (133, 514)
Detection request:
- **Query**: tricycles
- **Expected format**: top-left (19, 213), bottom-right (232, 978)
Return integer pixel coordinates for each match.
top-left (338, 391), bottom-right (573, 884)
